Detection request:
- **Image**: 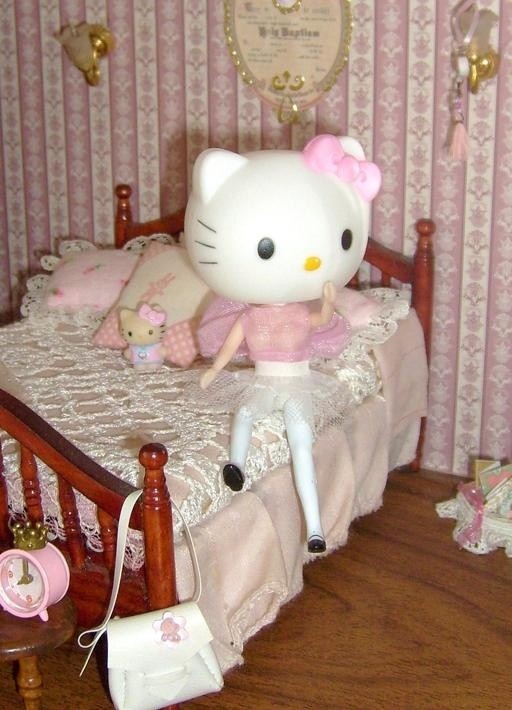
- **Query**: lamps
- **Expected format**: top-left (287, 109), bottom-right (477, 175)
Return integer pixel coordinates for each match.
top-left (52, 16), bottom-right (121, 90)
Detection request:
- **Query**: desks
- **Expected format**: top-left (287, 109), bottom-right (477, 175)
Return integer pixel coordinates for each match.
top-left (0, 591), bottom-right (81, 710)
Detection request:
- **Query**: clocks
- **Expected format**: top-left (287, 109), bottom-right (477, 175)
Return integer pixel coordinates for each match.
top-left (0, 518), bottom-right (72, 623)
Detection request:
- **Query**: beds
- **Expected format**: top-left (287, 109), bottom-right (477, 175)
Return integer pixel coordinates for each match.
top-left (0, 182), bottom-right (437, 628)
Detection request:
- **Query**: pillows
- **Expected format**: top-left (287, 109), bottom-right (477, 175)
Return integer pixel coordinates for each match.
top-left (20, 229), bottom-right (178, 330)
top-left (90, 238), bottom-right (220, 372)
